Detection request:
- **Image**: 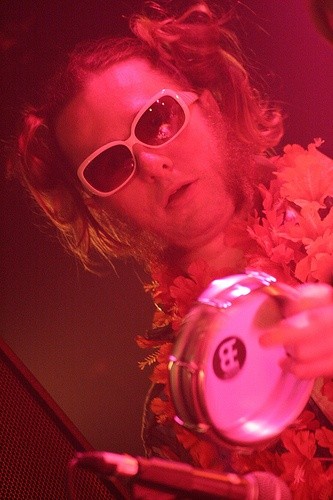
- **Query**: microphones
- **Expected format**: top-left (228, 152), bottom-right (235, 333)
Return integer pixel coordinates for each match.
top-left (74, 450), bottom-right (292, 500)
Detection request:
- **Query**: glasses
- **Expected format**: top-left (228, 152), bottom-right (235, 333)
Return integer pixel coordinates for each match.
top-left (77, 88), bottom-right (197, 199)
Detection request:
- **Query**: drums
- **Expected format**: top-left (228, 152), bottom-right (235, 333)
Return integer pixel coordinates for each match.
top-left (169, 273), bottom-right (315, 448)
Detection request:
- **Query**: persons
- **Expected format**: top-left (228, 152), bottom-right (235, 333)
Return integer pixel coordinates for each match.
top-left (8, 1), bottom-right (333, 500)
